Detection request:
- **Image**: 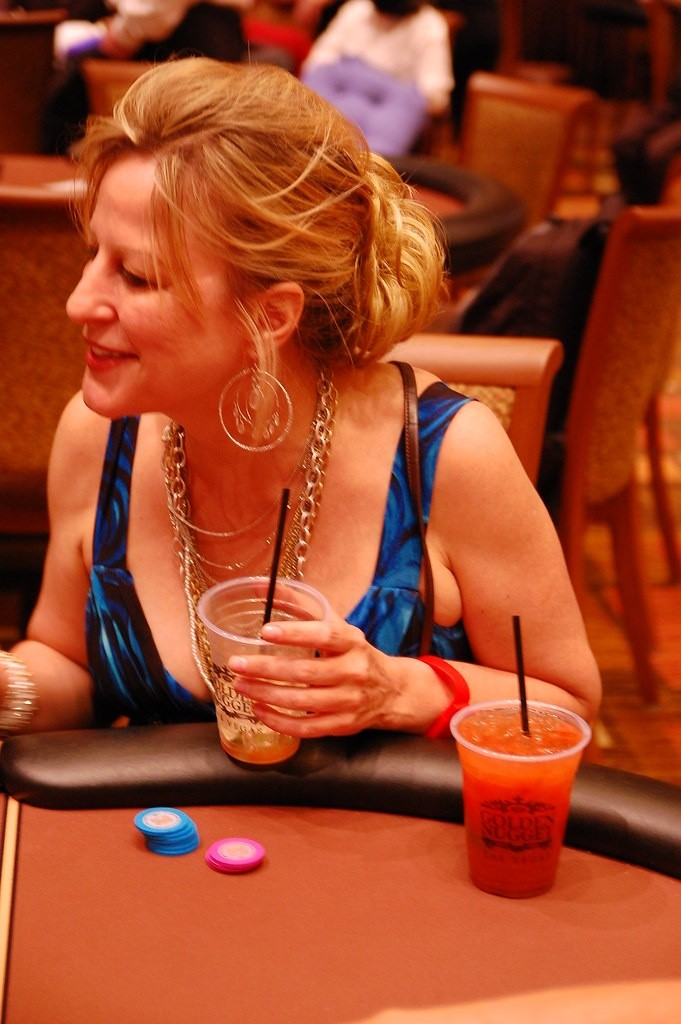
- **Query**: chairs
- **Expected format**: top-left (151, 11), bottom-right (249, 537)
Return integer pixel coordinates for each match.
top-left (538, 202), bottom-right (681, 707)
top-left (449, 70), bottom-right (600, 298)
top-left (366, 334), bottom-right (563, 495)
top-left (83, 58), bottom-right (164, 119)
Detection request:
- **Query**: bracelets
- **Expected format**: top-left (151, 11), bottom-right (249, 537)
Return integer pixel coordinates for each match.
top-left (1, 651), bottom-right (36, 736)
top-left (419, 653), bottom-right (470, 742)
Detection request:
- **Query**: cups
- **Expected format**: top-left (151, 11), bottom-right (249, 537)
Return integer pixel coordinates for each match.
top-left (451, 700), bottom-right (592, 899)
top-left (196, 575), bottom-right (327, 771)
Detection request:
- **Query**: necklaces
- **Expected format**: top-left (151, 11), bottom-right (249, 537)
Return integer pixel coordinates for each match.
top-left (162, 359), bottom-right (346, 694)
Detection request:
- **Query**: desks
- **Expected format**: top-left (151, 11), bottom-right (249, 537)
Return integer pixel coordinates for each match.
top-left (0, 152), bottom-right (527, 538)
top-left (0, 719), bottom-right (681, 1024)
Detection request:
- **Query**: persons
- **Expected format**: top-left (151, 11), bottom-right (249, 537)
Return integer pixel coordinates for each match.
top-left (1, 51), bottom-right (600, 752)
top-left (41, 0), bottom-right (244, 149)
top-left (299, 2), bottom-right (458, 148)
